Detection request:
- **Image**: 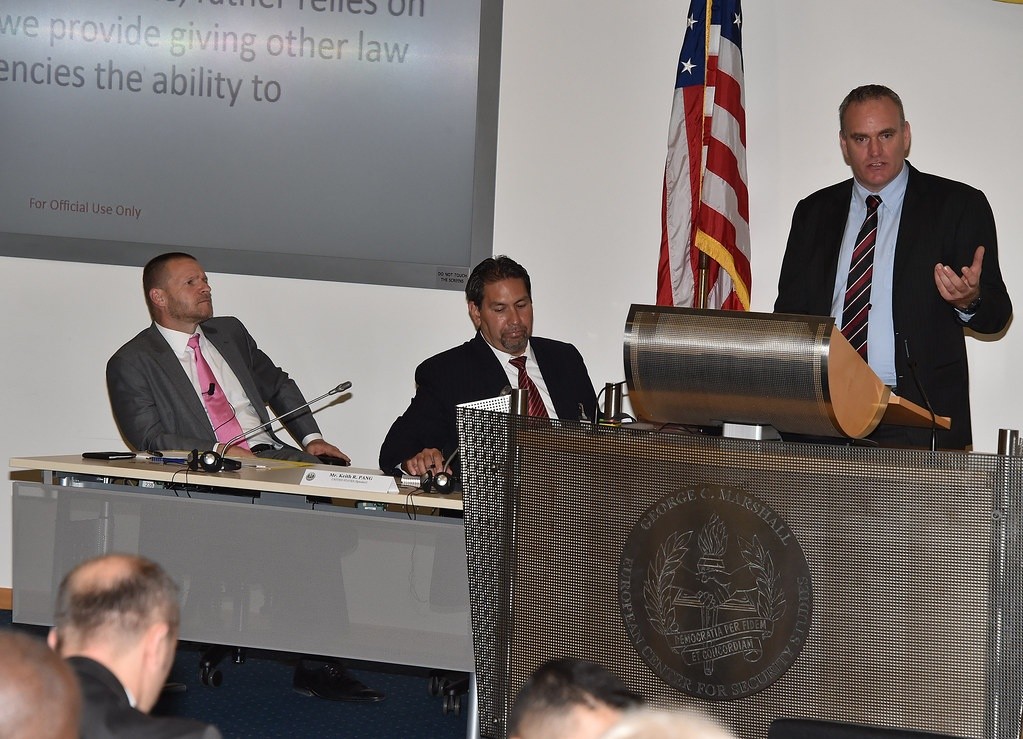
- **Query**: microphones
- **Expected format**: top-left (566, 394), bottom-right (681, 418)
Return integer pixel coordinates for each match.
top-left (220, 381), bottom-right (352, 458)
top-left (905, 356), bottom-right (938, 452)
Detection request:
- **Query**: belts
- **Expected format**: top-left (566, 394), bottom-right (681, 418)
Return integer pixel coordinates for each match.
top-left (249, 443), bottom-right (275, 454)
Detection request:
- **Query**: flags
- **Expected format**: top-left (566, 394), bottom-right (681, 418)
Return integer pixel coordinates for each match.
top-left (655, 0), bottom-right (752, 312)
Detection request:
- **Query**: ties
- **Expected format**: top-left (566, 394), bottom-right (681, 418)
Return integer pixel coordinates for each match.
top-left (509, 356), bottom-right (551, 429)
top-left (188, 333), bottom-right (251, 455)
top-left (840, 195), bottom-right (883, 364)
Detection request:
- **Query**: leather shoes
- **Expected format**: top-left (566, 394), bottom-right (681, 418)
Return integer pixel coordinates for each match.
top-left (293, 660), bottom-right (385, 702)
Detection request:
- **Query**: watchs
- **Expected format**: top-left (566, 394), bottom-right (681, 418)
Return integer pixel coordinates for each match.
top-left (956, 298), bottom-right (981, 314)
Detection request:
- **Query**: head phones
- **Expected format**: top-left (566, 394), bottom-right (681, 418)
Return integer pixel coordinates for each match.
top-left (420, 471), bottom-right (462, 494)
top-left (187, 449), bottom-right (241, 473)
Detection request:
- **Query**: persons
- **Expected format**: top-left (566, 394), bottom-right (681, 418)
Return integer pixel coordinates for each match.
top-left (378, 257), bottom-right (599, 475)
top-left (505, 658), bottom-right (729, 739)
top-left (106, 252), bottom-right (382, 702)
top-left (0, 551), bottom-right (221, 739)
top-left (773, 85), bottom-right (1013, 449)
